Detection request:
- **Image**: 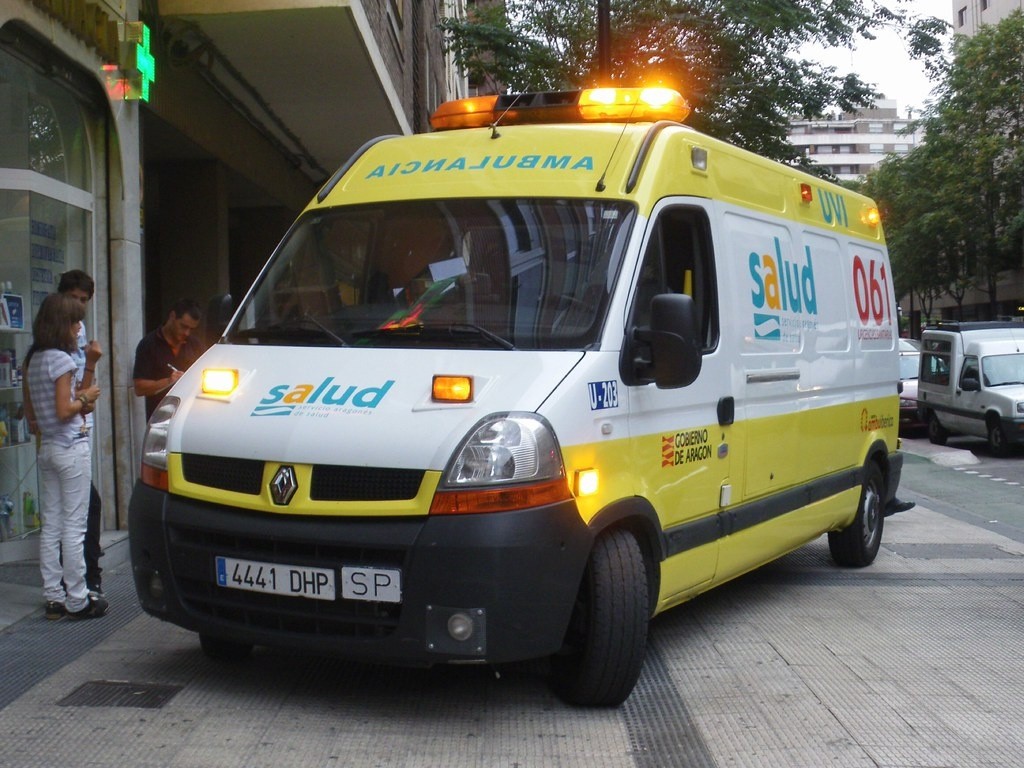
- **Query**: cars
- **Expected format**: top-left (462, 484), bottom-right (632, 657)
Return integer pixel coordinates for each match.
top-left (896, 337), bottom-right (950, 439)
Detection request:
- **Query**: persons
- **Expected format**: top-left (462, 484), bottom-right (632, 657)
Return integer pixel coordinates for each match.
top-left (22, 269), bottom-right (110, 621)
top-left (133, 297), bottom-right (207, 422)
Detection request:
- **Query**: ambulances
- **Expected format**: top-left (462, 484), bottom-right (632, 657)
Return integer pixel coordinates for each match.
top-left (130, 87), bottom-right (917, 714)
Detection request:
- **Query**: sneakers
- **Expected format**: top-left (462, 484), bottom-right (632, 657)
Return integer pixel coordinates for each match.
top-left (46, 600), bottom-right (67, 619)
top-left (65, 597), bottom-right (109, 621)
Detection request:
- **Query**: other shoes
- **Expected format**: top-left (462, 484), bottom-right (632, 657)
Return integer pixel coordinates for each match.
top-left (883, 496), bottom-right (916, 516)
top-left (88, 584), bottom-right (105, 599)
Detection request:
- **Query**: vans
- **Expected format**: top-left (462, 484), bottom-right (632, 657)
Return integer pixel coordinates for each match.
top-left (917, 319), bottom-right (1024, 460)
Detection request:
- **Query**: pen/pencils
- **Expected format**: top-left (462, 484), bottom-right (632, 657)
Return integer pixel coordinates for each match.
top-left (167, 364), bottom-right (178, 371)
